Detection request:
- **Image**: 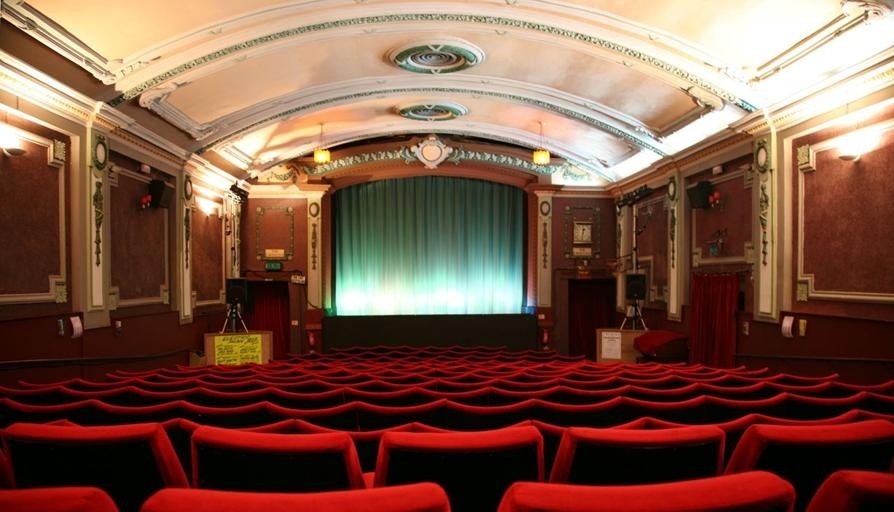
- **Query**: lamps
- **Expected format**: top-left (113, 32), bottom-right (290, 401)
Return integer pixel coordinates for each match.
top-left (314, 122), bottom-right (331, 164)
top-left (533, 121), bottom-right (550, 165)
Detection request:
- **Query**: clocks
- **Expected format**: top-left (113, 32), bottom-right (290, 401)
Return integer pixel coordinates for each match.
top-left (574, 221), bottom-right (593, 244)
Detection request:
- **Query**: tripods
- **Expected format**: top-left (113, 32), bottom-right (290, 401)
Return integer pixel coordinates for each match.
top-left (619, 300), bottom-right (648, 330)
top-left (220, 304), bottom-right (249, 336)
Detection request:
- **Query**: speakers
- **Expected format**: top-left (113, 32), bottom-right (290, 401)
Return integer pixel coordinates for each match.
top-left (226, 278), bottom-right (248, 303)
top-left (626, 274), bottom-right (646, 300)
top-left (696, 181), bottom-right (712, 208)
top-left (149, 180), bottom-right (165, 208)
